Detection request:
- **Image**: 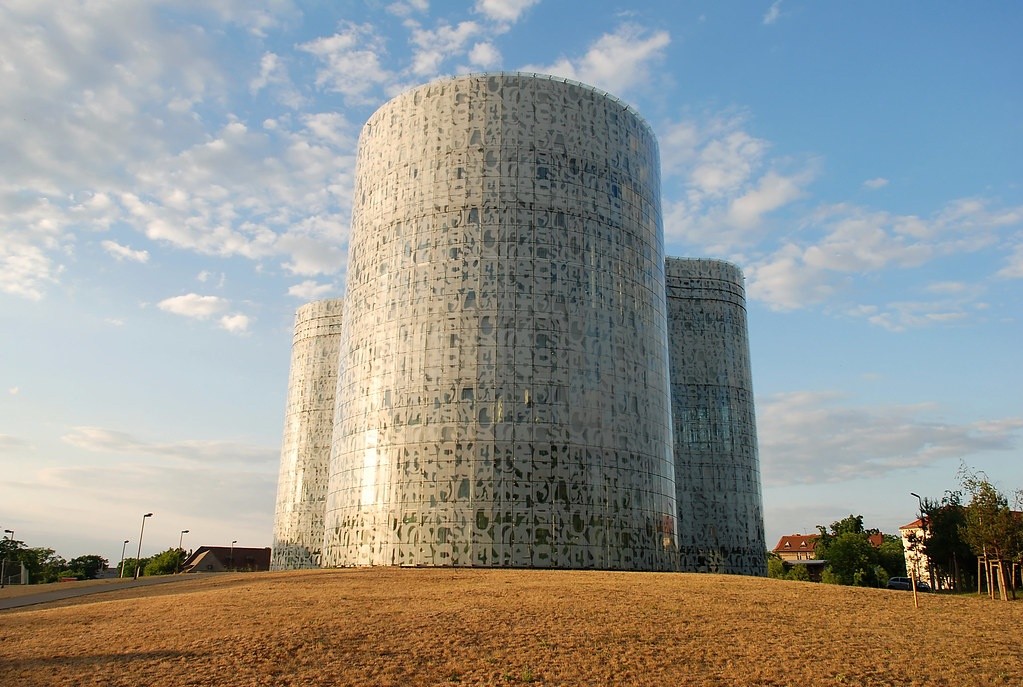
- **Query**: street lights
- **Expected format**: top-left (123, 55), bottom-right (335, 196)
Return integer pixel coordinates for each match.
top-left (4, 529), bottom-right (15, 541)
top-left (910, 492), bottom-right (933, 589)
top-left (175, 530), bottom-right (190, 573)
top-left (230, 540), bottom-right (237, 567)
top-left (119, 540), bottom-right (130, 578)
top-left (134, 512), bottom-right (153, 580)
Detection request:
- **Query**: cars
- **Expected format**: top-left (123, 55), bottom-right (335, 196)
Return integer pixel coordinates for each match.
top-left (886, 576), bottom-right (912, 591)
